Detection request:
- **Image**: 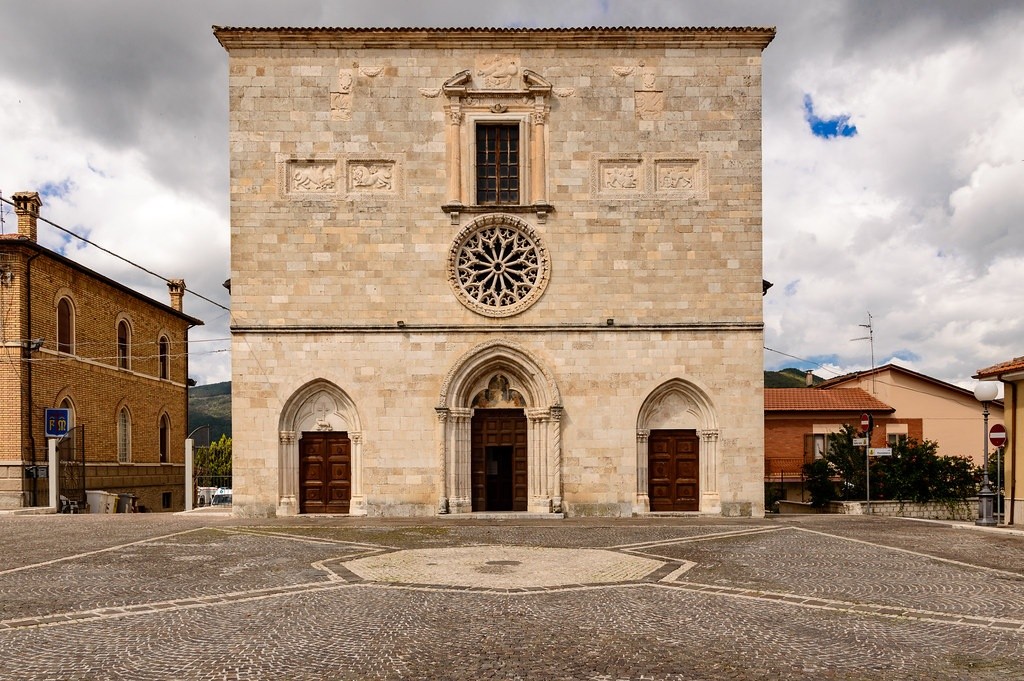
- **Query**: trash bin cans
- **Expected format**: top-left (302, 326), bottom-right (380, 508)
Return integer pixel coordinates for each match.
top-left (119, 492), bottom-right (139, 514)
top-left (84, 489), bottom-right (121, 514)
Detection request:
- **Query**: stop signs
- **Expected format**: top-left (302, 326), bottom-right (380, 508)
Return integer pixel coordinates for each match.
top-left (989, 424), bottom-right (1006, 447)
top-left (860, 414), bottom-right (869, 432)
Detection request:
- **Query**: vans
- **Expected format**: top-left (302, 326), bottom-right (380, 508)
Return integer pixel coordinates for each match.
top-left (210, 488), bottom-right (232, 507)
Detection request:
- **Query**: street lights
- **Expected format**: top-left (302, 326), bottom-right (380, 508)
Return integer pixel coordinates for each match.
top-left (974, 381), bottom-right (998, 527)
